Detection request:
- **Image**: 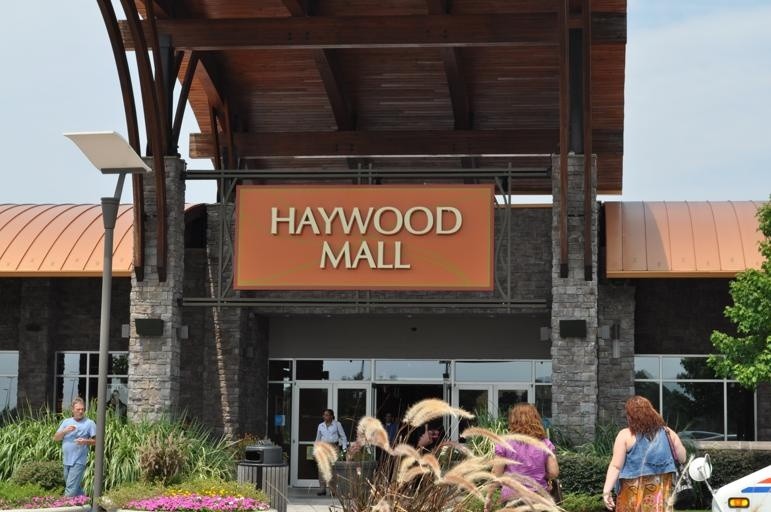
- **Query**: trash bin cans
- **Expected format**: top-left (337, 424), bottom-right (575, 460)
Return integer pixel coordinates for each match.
top-left (237, 445), bottom-right (289, 512)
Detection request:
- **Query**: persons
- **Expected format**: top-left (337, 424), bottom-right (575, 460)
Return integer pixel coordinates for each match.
top-left (412, 415), bottom-right (446, 492)
top-left (51, 394), bottom-right (97, 498)
top-left (311, 409), bottom-right (347, 498)
top-left (378, 412), bottom-right (399, 484)
top-left (105, 388), bottom-right (127, 429)
top-left (482, 401), bottom-right (561, 512)
top-left (601, 395), bottom-right (688, 512)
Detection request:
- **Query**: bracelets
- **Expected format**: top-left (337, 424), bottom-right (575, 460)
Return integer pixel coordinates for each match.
top-left (60, 429), bottom-right (66, 435)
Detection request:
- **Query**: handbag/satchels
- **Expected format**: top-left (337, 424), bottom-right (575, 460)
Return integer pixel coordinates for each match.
top-left (548, 478), bottom-right (563, 506)
top-left (671, 463), bottom-right (696, 510)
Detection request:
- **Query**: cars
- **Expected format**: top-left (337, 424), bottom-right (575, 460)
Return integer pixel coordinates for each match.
top-left (677, 431), bottom-right (738, 441)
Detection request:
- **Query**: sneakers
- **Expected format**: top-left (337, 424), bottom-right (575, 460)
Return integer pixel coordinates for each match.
top-left (317, 490), bottom-right (326, 496)
